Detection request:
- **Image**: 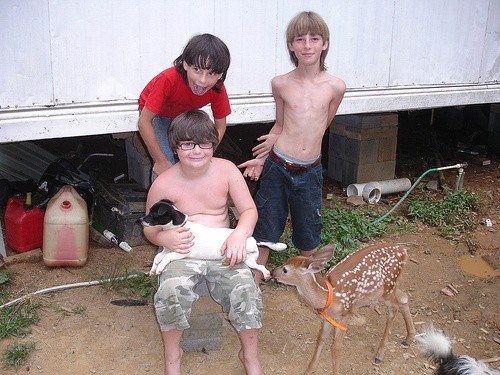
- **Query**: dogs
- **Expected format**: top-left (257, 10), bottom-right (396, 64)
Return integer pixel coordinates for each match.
top-left (411, 321), bottom-right (500, 375)
top-left (138, 199), bottom-right (290, 282)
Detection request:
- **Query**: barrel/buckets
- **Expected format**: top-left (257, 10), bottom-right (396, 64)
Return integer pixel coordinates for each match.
top-left (42, 184), bottom-right (89, 268)
top-left (5, 194), bottom-right (44, 254)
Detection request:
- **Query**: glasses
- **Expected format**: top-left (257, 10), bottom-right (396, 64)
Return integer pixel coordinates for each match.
top-left (178, 141), bottom-right (215, 150)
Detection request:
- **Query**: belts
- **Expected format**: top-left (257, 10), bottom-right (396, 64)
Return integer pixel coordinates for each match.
top-left (269, 149), bottom-right (321, 172)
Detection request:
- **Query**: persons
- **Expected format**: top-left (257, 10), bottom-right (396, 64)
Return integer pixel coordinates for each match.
top-left (138, 33), bottom-right (231, 184)
top-left (236, 10), bottom-right (346, 285)
top-left (144, 110), bottom-right (266, 375)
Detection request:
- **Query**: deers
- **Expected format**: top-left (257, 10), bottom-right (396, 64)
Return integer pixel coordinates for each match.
top-left (269, 236), bottom-right (419, 375)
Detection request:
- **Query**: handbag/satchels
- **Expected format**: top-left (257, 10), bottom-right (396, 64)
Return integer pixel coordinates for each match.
top-left (37, 159), bottom-right (96, 211)
